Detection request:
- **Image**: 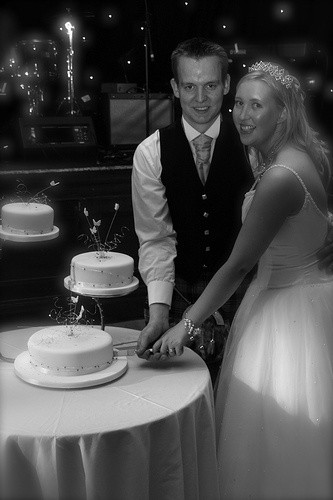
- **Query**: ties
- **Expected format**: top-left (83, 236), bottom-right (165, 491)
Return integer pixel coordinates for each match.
top-left (193, 134), bottom-right (213, 184)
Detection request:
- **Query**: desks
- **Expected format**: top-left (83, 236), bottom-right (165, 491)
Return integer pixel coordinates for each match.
top-left (0, 324), bottom-right (216, 500)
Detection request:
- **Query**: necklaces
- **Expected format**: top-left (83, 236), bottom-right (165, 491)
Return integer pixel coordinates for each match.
top-left (259, 149), bottom-right (275, 171)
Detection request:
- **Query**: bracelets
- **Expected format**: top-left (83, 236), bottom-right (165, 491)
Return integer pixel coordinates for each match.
top-left (184, 318), bottom-right (200, 337)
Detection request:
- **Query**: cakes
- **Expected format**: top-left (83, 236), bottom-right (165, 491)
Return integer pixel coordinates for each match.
top-left (1, 202), bottom-right (54, 235)
top-left (28, 324), bottom-right (113, 376)
top-left (69, 251), bottom-right (134, 288)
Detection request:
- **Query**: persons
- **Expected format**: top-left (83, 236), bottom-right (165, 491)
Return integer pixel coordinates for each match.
top-left (130, 39), bottom-right (255, 382)
top-left (153, 62), bottom-right (333, 500)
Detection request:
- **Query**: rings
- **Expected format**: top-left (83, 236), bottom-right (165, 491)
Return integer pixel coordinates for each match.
top-left (168, 348), bottom-right (175, 353)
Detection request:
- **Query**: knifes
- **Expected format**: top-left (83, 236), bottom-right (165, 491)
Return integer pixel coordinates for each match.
top-left (112, 346), bottom-right (184, 357)
